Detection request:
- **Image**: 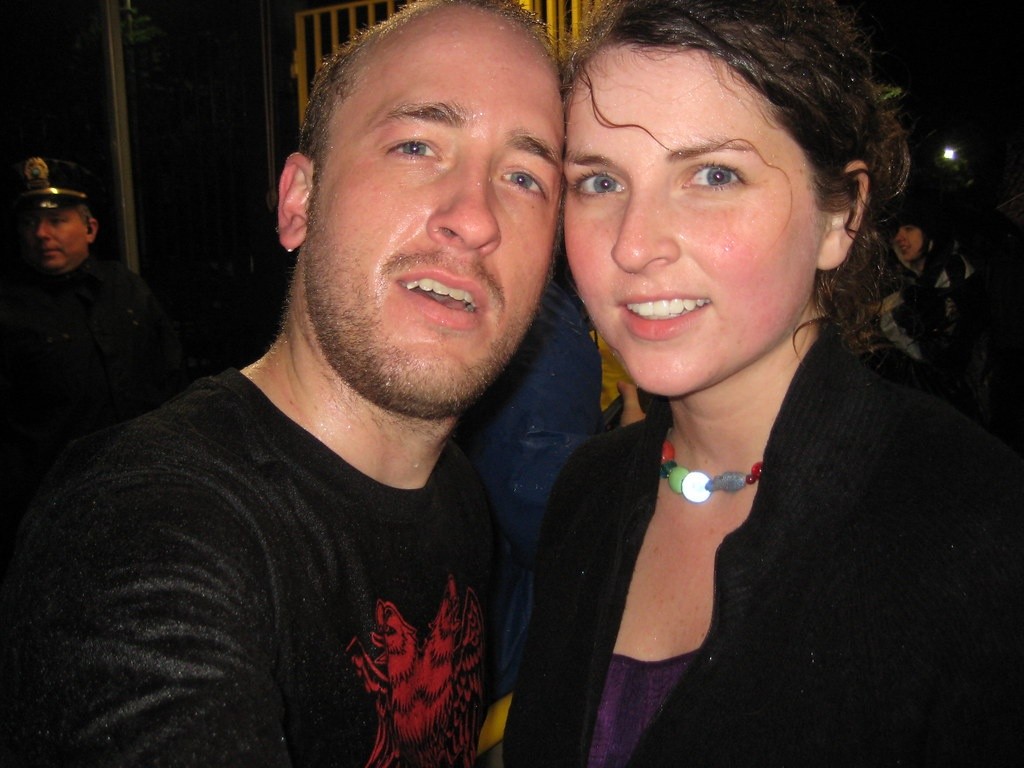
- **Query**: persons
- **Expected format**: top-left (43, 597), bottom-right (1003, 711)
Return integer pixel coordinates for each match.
top-left (0, 157), bottom-right (1024, 768)
top-left (496, 1), bottom-right (1024, 768)
top-left (0, 0), bottom-right (563, 768)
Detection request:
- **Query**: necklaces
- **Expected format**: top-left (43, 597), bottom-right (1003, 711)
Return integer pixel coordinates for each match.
top-left (658, 434), bottom-right (766, 503)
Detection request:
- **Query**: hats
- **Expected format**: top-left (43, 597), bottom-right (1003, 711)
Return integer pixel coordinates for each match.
top-left (886, 190), bottom-right (937, 240)
top-left (5, 156), bottom-right (98, 217)
